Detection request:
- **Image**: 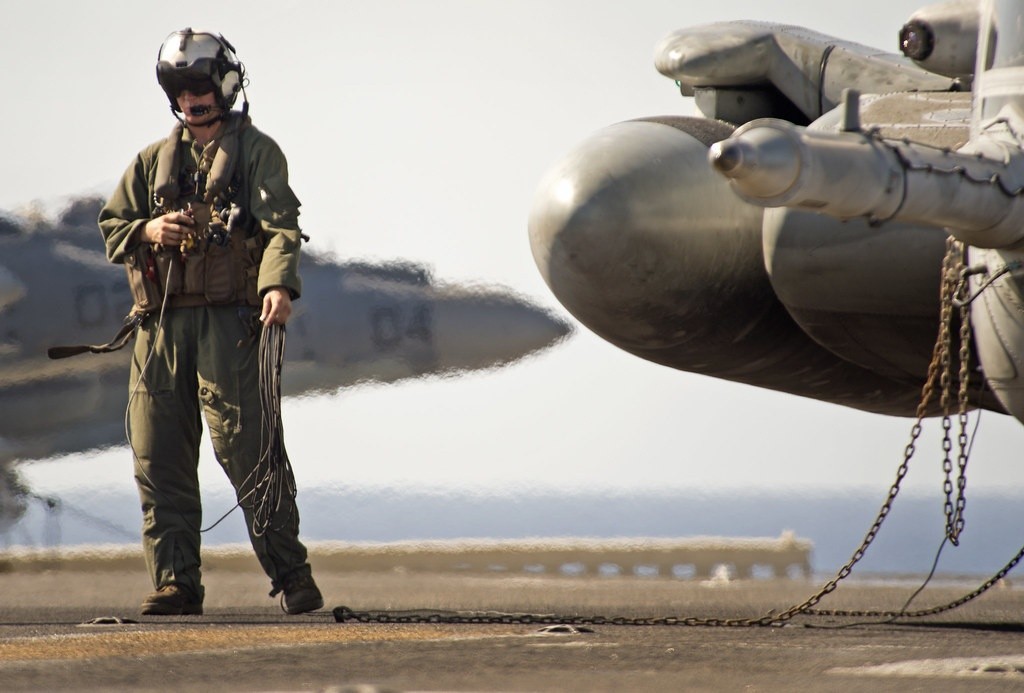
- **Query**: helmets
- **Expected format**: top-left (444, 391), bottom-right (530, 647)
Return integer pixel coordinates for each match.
top-left (156, 27), bottom-right (244, 112)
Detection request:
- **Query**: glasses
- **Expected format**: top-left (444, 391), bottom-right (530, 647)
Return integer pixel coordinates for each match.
top-left (170, 77), bottom-right (216, 96)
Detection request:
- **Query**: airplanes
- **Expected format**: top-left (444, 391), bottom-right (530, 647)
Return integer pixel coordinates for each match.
top-left (522, 1), bottom-right (1024, 427)
top-left (0, 194), bottom-right (579, 547)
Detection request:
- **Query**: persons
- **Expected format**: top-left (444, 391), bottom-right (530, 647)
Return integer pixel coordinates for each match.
top-left (99, 21), bottom-right (326, 616)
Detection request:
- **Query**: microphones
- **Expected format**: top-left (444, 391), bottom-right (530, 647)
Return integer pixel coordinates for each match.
top-left (190, 84), bottom-right (241, 116)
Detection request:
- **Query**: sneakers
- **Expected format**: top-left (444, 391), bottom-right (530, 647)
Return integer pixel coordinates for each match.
top-left (139, 584), bottom-right (205, 615)
top-left (282, 564), bottom-right (324, 614)
